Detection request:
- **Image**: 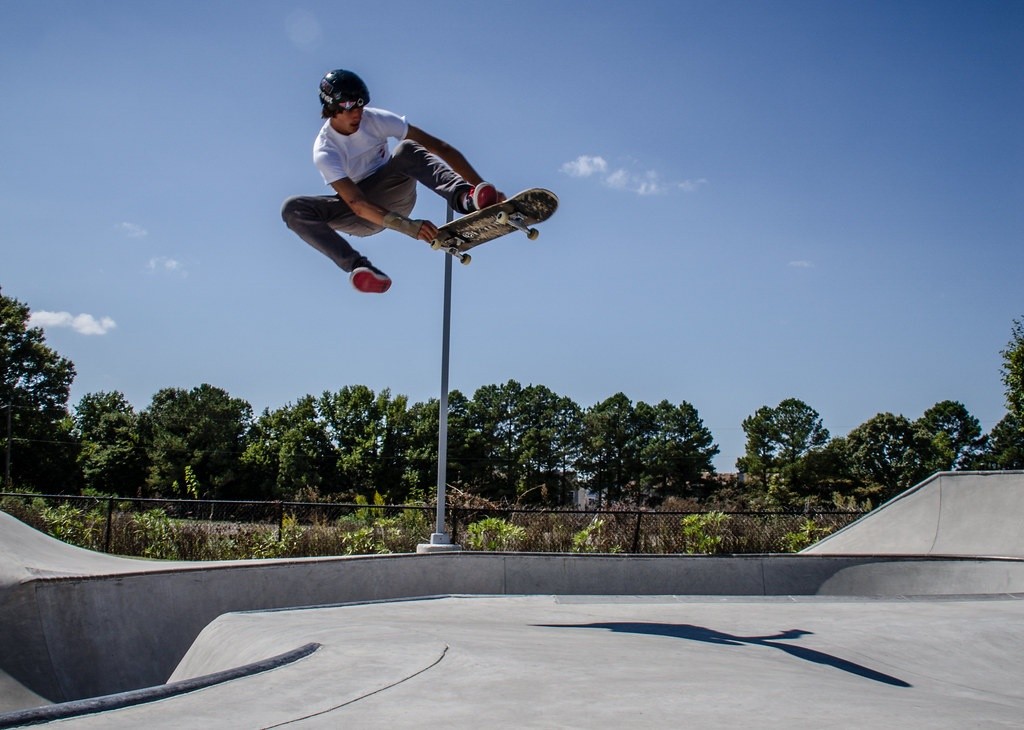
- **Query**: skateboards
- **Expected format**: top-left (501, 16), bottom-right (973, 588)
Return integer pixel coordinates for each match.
top-left (429, 187), bottom-right (560, 264)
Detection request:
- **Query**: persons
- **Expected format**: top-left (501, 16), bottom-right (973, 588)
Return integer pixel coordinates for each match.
top-left (280, 67), bottom-right (507, 294)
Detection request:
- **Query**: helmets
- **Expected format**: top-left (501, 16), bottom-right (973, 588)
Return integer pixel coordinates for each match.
top-left (318, 70), bottom-right (370, 110)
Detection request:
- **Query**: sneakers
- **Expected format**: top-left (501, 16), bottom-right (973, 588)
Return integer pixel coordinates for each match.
top-left (350, 256), bottom-right (392, 293)
top-left (463, 181), bottom-right (497, 214)
